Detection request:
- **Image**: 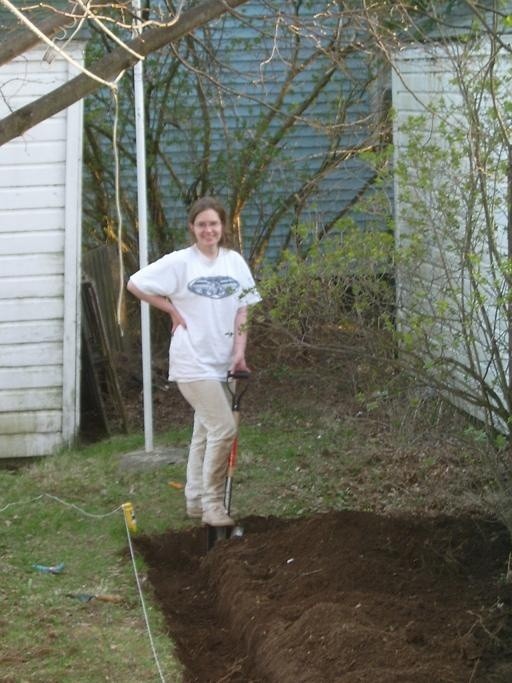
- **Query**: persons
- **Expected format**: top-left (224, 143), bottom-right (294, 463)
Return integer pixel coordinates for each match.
top-left (126, 198), bottom-right (262, 527)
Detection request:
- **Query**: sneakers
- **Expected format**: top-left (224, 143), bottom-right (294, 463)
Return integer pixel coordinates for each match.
top-left (186, 507), bottom-right (203, 518)
top-left (202, 511), bottom-right (234, 527)
